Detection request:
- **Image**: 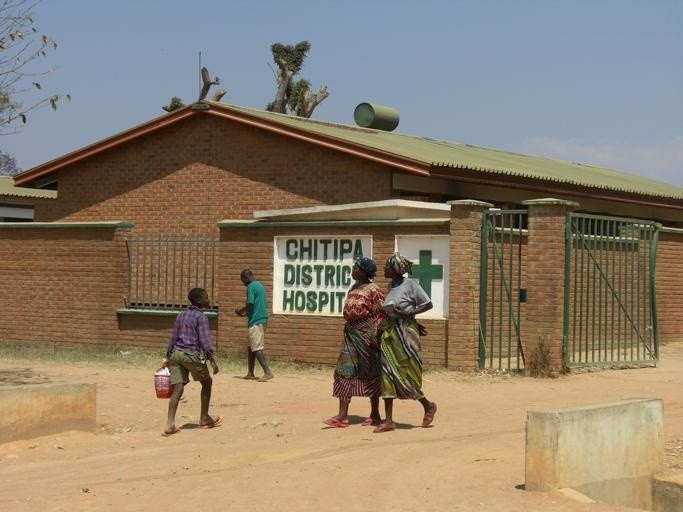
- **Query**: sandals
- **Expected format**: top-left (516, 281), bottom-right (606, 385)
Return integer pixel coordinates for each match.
top-left (374, 423), bottom-right (395, 432)
top-left (423, 403), bottom-right (437, 428)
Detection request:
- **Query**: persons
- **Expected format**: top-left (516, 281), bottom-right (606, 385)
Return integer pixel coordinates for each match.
top-left (372, 252), bottom-right (438, 433)
top-left (321, 258), bottom-right (387, 428)
top-left (159, 288), bottom-right (224, 437)
top-left (233, 268), bottom-right (275, 382)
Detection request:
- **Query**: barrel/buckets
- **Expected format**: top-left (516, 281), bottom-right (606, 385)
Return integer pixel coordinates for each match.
top-left (354, 101), bottom-right (399, 131)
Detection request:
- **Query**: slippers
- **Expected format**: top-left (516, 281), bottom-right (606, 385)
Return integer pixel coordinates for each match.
top-left (324, 416), bottom-right (349, 427)
top-left (362, 417), bottom-right (380, 425)
top-left (162, 426), bottom-right (181, 436)
top-left (200, 415), bottom-right (224, 429)
